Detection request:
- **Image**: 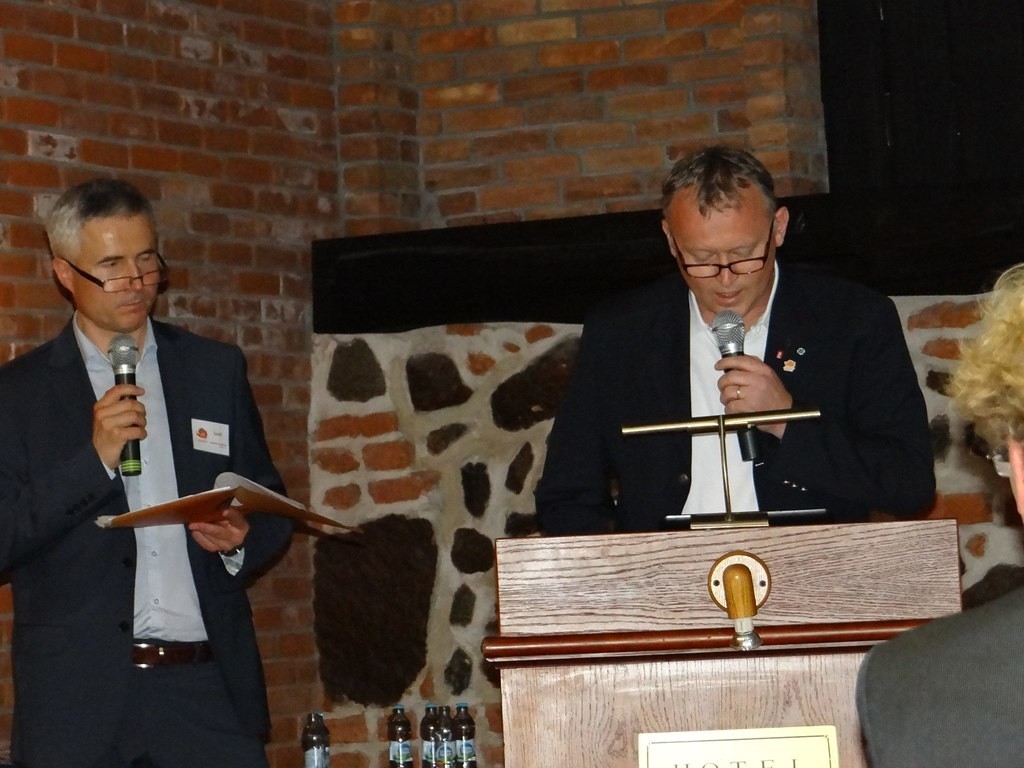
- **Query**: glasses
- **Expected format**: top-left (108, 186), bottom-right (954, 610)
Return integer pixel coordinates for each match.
top-left (986, 432), bottom-right (1017, 478)
top-left (673, 217), bottom-right (775, 279)
top-left (58, 253), bottom-right (171, 292)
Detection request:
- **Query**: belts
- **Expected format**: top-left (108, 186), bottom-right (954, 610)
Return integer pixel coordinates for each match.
top-left (132, 642), bottom-right (215, 666)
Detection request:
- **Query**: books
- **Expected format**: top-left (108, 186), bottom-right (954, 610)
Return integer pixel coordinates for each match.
top-left (95, 472), bottom-right (364, 533)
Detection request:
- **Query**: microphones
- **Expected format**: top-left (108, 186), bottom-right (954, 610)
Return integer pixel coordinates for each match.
top-left (711, 310), bottom-right (762, 462)
top-left (107, 334), bottom-right (142, 476)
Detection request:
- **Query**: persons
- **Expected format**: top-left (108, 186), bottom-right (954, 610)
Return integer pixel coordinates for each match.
top-left (855, 264), bottom-right (1024, 768)
top-left (0, 178), bottom-right (290, 768)
top-left (535, 142), bottom-right (938, 537)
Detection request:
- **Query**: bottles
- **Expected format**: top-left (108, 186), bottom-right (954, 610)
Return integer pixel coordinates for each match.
top-left (388, 704), bottom-right (413, 768)
top-left (420, 704), bottom-right (439, 768)
top-left (438, 706), bottom-right (456, 768)
top-left (454, 703), bottom-right (477, 768)
top-left (302, 712), bottom-right (330, 768)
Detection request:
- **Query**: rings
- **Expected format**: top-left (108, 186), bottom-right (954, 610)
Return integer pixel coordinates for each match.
top-left (736, 384), bottom-right (741, 399)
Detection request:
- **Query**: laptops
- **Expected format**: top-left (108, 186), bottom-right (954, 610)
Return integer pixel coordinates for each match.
top-left (665, 509), bottom-right (831, 530)
